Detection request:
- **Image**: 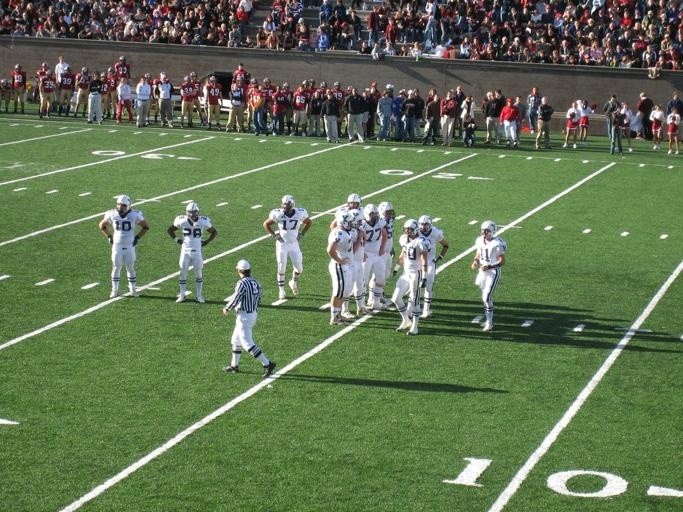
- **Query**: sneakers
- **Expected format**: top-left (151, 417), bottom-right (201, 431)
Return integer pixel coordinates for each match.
top-left (222, 365), bottom-right (240, 374)
top-left (176, 294), bottom-right (186, 303)
top-left (261, 360), bottom-right (276, 379)
top-left (480, 320), bottom-right (494, 332)
top-left (109, 288), bottom-right (121, 298)
top-left (288, 280), bottom-right (300, 295)
top-left (357, 297), bottom-right (394, 316)
top-left (329, 316), bottom-right (350, 327)
top-left (406, 324), bottom-right (419, 335)
top-left (340, 309), bottom-right (356, 319)
top-left (396, 319), bottom-right (413, 332)
top-left (278, 286), bottom-right (286, 300)
top-left (419, 310), bottom-right (433, 319)
top-left (130, 288), bottom-right (140, 297)
top-left (196, 295), bottom-right (205, 304)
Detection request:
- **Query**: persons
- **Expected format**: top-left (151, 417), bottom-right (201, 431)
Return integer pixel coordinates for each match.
top-left (180, 72), bottom-right (223, 130)
top-left (227, 63), bottom-right (476, 148)
top-left (0, 55), bottom-right (173, 128)
top-left (99, 195), bottom-right (150, 297)
top-left (602, 90), bottom-right (681, 153)
top-left (482, 86), bottom-right (554, 151)
top-left (562, 99), bottom-right (595, 149)
top-left (167, 201), bottom-right (216, 303)
top-left (470, 220), bottom-right (506, 331)
top-left (317, 192), bottom-right (448, 334)
top-left (262, 194), bottom-right (312, 299)
top-left (221, 259), bottom-right (276, 378)
top-left (1, 1), bottom-right (255, 48)
top-left (255, 1), bottom-right (681, 71)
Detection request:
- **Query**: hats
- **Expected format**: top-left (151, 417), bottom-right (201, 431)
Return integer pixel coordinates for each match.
top-left (235, 259), bottom-right (251, 271)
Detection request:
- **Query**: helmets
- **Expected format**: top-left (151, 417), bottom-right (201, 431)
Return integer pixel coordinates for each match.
top-left (419, 215), bottom-right (433, 227)
top-left (282, 194), bottom-right (295, 209)
top-left (8, 55), bottom-right (126, 86)
top-left (186, 202), bottom-right (200, 217)
top-left (336, 208), bottom-right (364, 226)
top-left (378, 201), bottom-right (394, 219)
top-left (143, 72), bottom-right (290, 90)
top-left (116, 194), bottom-right (132, 212)
top-left (303, 79), bottom-right (414, 95)
top-left (481, 220), bottom-right (497, 237)
top-left (403, 219), bottom-right (420, 236)
top-left (347, 193), bottom-right (361, 208)
top-left (363, 203), bottom-right (380, 222)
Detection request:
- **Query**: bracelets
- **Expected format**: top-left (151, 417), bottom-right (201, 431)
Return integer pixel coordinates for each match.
top-left (173, 236), bottom-right (180, 243)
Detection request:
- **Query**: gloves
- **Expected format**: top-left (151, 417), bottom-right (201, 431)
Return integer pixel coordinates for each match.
top-left (107, 234), bottom-right (113, 245)
top-left (420, 278), bottom-right (427, 288)
top-left (390, 247), bottom-right (395, 258)
top-left (392, 270), bottom-right (398, 277)
top-left (174, 237), bottom-right (183, 245)
top-left (133, 235), bottom-right (141, 247)
top-left (201, 240), bottom-right (208, 247)
top-left (433, 254), bottom-right (444, 264)
top-left (272, 234), bottom-right (285, 243)
top-left (471, 261), bottom-right (478, 273)
top-left (296, 231), bottom-right (302, 243)
top-left (480, 263), bottom-right (492, 272)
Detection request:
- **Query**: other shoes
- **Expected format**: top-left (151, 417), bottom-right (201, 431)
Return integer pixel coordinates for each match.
top-left (6, 108), bottom-right (151, 128)
top-left (159, 123), bottom-right (324, 137)
top-left (327, 129), bottom-right (553, 150)
